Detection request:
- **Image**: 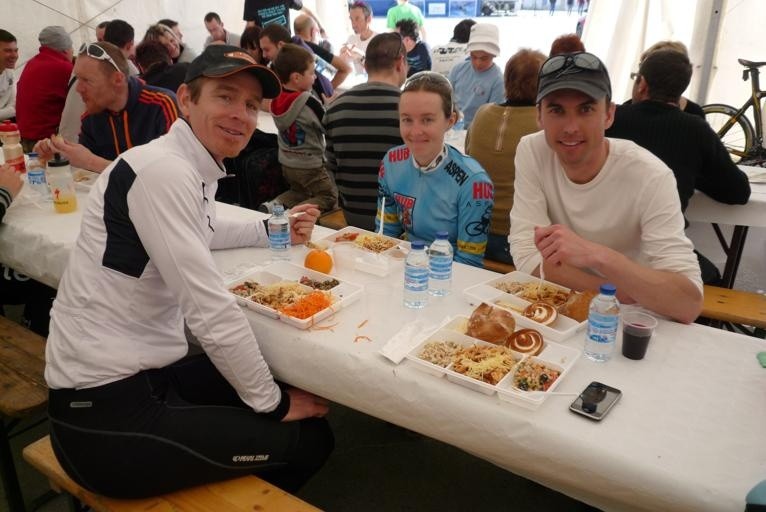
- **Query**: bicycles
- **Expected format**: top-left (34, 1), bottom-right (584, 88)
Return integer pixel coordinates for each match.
top-left (695, 57), bottom-right (766, 168)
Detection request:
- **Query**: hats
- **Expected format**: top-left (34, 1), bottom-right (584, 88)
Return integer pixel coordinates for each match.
top-left (184, 44), bottom-right (282, 99)
top-left (38, 26), bottom-right (74, 52)
top-left (535, 52), bottom-right (611, 103)
top-left (467, 23), bottom-right (502, 58)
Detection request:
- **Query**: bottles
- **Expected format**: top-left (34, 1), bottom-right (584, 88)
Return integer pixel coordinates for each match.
top-left (425, 231), bottom-right (456, 298)
top-left (310, 53), bottom-right (339, 82)
top-left (266, 202), bottom-right (293, 263)
top-left (583, 282), bottom-right (620, 364)
top-left (26, 151), bottom-right (49, 198)
top-left (0, 120), bottom-right (25, 174)
top-left (403, 237), bottom-right (430, 309)
top-left (45, 152), bottom-right (78, 215)
top-left (343, 42), bottom-right (366, 65)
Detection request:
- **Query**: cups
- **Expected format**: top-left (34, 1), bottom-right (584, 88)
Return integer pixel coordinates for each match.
top-left (330, 241), bottom-right (358, 284)
top-left (619, 312), bottom-right (659, 362)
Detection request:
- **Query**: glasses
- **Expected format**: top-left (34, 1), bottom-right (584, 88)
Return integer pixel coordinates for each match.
top-left (76, 41), bottom-right (122, 73)
top-left (537, 53), bottom-right (610, 92)
top-left (630, 72), bottom-right (641, 81)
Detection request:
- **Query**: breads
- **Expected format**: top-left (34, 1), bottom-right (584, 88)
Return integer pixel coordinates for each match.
top-left (467, 302), bottom-right (515, 346)
top-left (506, 328), bottom-right (544, 356)
top-left (523, 301), bottom-right (559, 326)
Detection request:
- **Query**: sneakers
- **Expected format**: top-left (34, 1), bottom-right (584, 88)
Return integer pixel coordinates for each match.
top-left (259, 201), bottom-right (275, 215)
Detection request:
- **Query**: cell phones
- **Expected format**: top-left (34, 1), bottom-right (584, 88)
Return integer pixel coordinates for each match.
top-left (569, 381), bottom-right (622, 422)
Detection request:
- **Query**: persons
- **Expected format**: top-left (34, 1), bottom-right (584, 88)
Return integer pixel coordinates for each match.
top-left (40, 47), bottom-right (335, 493)
